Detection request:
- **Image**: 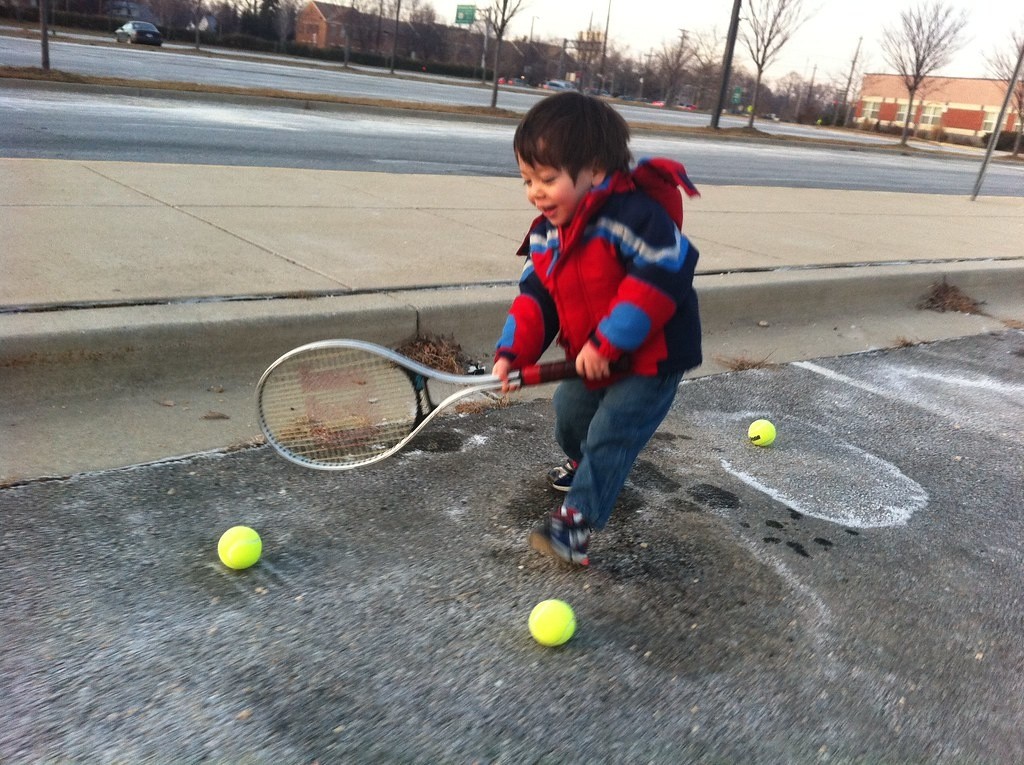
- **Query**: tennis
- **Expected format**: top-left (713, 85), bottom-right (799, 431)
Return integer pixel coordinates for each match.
top-left (528, 598), bottom-right (577, 645)
top-left (218, 526), bottom-right (262, 569)
top-left (748, 418), bottom-right (776, 446)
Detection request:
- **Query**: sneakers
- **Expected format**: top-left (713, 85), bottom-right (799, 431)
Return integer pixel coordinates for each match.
top-left (529, 506), bottom-right (590, 566)
top-left (546, 459), bottom-right (578, 492)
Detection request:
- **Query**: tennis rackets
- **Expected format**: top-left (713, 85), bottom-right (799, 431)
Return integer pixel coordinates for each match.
top-left (257, 340), bottom-right (577, 470)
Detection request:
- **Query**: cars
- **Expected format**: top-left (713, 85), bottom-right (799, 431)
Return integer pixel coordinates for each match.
top-left (497, 77), bottom-right (654, 104)
top-left (115, 21), bottom-right (163, 46)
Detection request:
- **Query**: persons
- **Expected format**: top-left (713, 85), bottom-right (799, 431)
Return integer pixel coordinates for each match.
top-left (491, 91), bottom-right (703, 565)
